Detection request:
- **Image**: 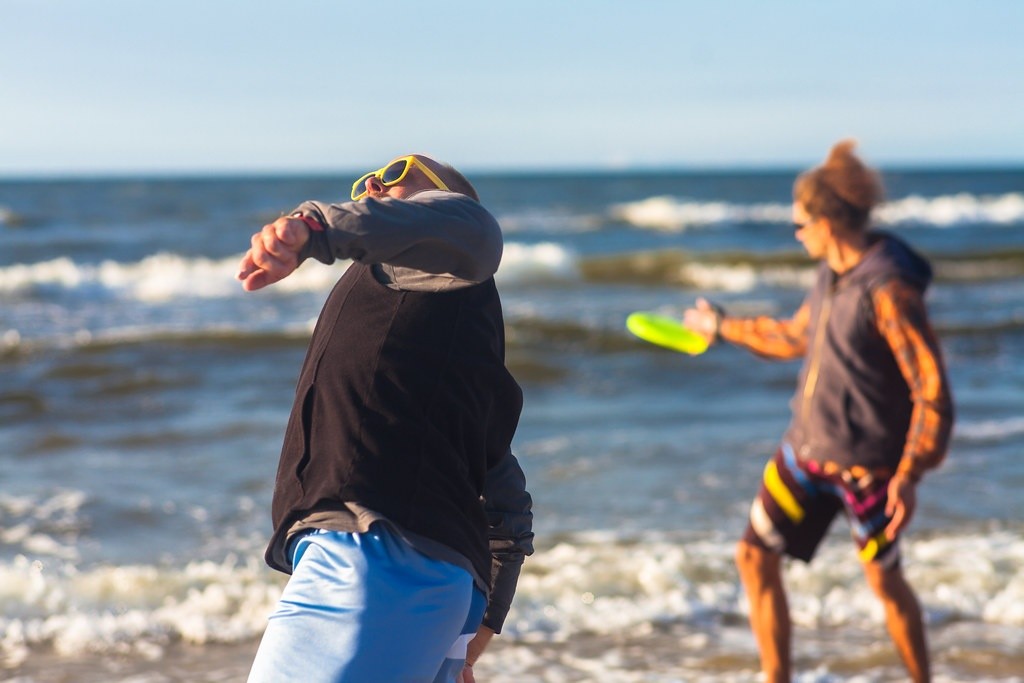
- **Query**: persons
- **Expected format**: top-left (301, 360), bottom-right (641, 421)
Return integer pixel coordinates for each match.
top-left (681, 138), bottom-right (955, 682)
top-left (234, 152), bottom-right (537, 683)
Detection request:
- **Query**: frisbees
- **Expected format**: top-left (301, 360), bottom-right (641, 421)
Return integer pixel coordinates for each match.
top-left (623, 313), bottom-right (712, 358)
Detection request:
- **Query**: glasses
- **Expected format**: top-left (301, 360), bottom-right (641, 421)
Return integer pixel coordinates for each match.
top-left (351, 155), bottom-right (451, 202)
